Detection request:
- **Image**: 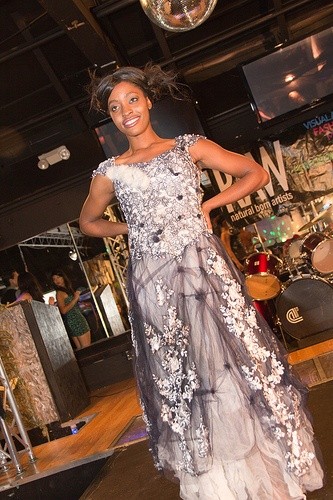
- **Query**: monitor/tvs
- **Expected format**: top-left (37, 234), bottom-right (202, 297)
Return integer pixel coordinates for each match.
top-left (237, 20), bottom-right (333, 131)
top-left (90, 85), bottom-right (212, 162)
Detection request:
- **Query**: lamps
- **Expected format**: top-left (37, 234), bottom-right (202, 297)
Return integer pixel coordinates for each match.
top-left (37, 146), bottom-right (70, 169)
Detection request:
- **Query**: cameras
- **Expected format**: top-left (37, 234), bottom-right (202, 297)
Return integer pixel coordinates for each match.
top-left (77, 287), bottom-right (85, 292)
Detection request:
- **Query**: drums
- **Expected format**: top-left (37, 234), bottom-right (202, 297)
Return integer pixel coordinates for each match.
top-left (299, 231), bottom-right (333, 274)
top-left (243, 252), bottom-right (281, 300)
top-left (275, 274), bottom-right (333, 340)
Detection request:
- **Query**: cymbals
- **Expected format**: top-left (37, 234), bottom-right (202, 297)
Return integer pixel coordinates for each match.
top-left (298, 206), bottom-right (333, 232)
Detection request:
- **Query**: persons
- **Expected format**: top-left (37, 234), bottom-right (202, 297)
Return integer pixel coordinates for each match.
top-left (15, 273), bottom-right (46, 303)
top-left (79, 62), bottom-right (324, 500)
top-left (0, 266), bottom-right (21, 304)
top-left (50, 268), bottom-right (90, 347)
